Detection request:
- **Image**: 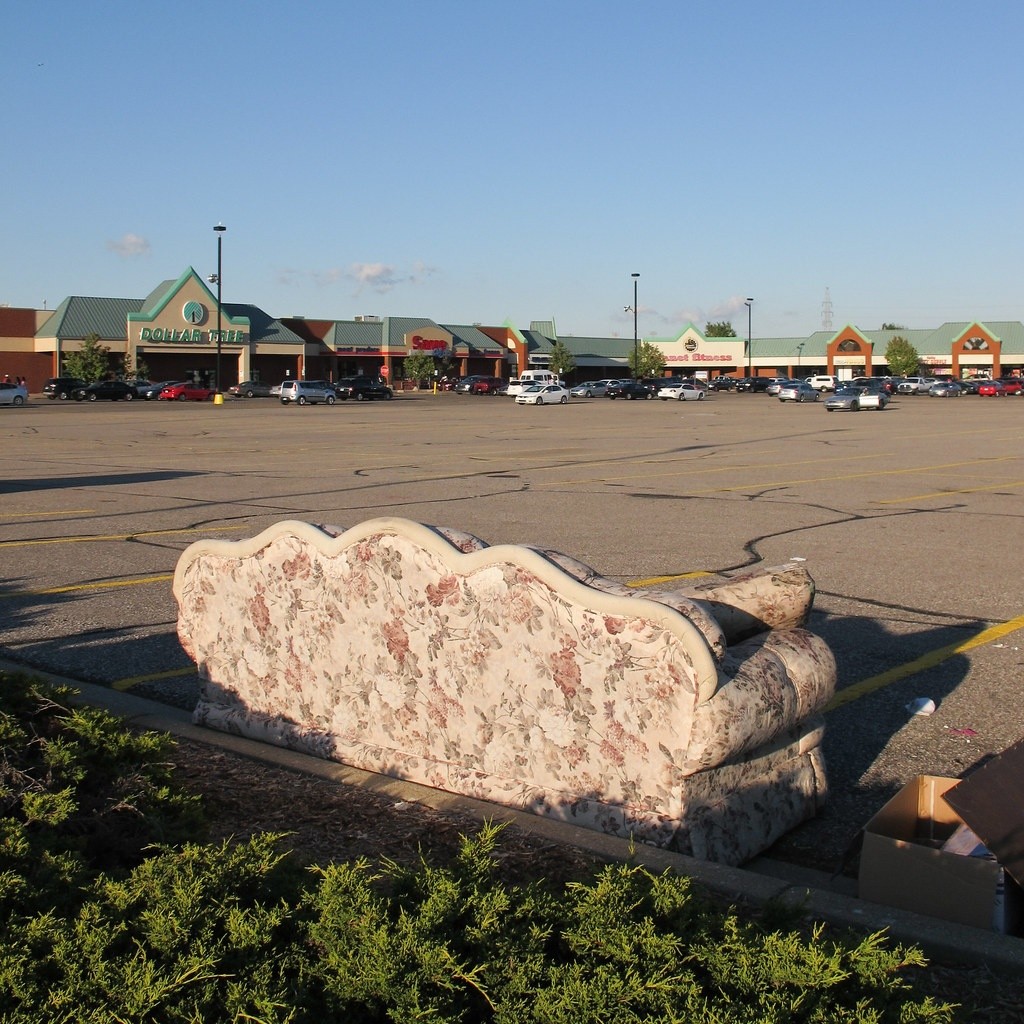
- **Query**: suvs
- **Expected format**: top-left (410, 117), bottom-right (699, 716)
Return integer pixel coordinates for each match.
top-left (335, 377), bottom-right (393, 401)
top-left (42, 377), bottom-right (90, 400)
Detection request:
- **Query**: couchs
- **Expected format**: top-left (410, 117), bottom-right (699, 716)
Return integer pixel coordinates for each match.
top-left (174, 518), bottom-right (838, 867)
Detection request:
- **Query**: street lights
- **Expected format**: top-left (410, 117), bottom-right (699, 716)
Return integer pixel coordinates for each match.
top-left (745, 297), bottom-right (754, 377)
top-left (212, 221), bottom-right (228, 393)
top-left (630, 272), bottom-right (641, 385)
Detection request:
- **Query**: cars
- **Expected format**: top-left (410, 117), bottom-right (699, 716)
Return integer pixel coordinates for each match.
top-left (0, 382), bottom-right (29, 407)
top-left (515, 384), bottom-right (569, 405)
top-left (570, 375), bottom-right (820, 402)
top-left (129, 380), bottom-right (213, 402)
top-left (281, 379), bottom-right (336, 405)
top-left (822, 386), bottom-right (888, 412)
top-left (437, 376), bottom-right (542, 399)
top-left (70, 381), bottom-right (137, 402)
top-left (229, 381), bottom-right (271, 397)
top-left (835, 372), bottom-right (1024, 398)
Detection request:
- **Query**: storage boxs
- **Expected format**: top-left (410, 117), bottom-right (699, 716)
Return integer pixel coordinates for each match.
top-left (851, 774), bottom-right (1024, 936)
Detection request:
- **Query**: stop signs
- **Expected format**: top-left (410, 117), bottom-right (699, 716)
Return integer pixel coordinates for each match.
top-left (380, 366), bottom-right (389, 376)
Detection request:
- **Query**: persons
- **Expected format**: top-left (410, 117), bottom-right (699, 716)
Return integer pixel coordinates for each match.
top-left (2, 375), bottom-right (11, 383)
top-left (14, 376), bottom-right (27, 386)
top-left (682, 375), bottom-right (693, 381)
top-left (862, 387), bottom-right (870, 411)
top-left (377, 372), bottom-right (384, 385)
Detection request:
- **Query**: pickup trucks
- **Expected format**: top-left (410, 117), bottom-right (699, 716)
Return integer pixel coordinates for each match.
top-left (272, 386), bottom-right (282, 395)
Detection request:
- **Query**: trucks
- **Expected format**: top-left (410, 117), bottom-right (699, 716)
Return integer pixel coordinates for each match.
top-left (523, 370), bottom-right (560, 386)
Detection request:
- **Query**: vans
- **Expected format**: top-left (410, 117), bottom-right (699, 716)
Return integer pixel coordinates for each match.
top-left (805, 375), bottom-right (840, 393)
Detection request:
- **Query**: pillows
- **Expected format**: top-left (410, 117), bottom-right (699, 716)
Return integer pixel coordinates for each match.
top-left (675, 564), bottom-right (815, 644)
